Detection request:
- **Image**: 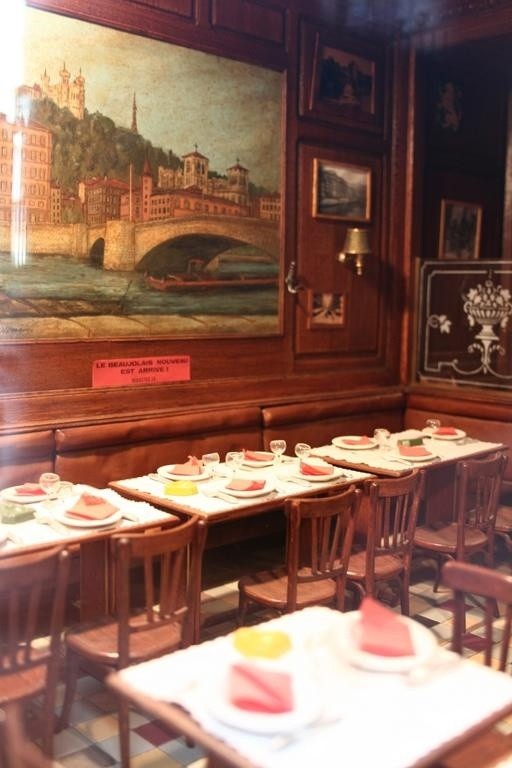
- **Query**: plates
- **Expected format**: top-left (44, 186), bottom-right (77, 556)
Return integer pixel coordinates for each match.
top-left (294, 463), bottom-right (345, 480)
top-left (329, 604), bottom-right (444, 675)
top-left (157, 462), bottom-right (212, 479)
top-left (237, 449), bottom-right (278, 467)
top-left (53, 495), bottom-right (125, 527)
top-left (3, 483), bottom-right (49, 502)
top-left (218, 474), bottom-right (276, 499)
top-left (332, 419), bottom-right (468, 461)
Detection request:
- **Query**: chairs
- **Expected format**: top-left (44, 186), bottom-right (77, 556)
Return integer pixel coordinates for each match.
top-left (2, 393), bottom-right (512, 765)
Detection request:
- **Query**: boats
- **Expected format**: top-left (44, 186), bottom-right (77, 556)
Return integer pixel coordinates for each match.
top-left (142, 258), bottom-right (280, 298)
top-left (323, 93), bottom-right (361, 107)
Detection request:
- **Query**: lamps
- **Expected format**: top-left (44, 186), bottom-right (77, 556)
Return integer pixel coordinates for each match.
top-left (336, 223), bottom-right (375, 279)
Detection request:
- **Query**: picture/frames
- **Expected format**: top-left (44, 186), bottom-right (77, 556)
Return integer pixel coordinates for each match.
top-left (310, 157), bottom-right (373, 223)
top-left (1, 0), bottom-right (288, 348)
top-left (436, 196), bottom-right (483, 261)
top-left (295, 16), bottom-right (388, 136)
top-left (307, 287), bottom-right (348, 330)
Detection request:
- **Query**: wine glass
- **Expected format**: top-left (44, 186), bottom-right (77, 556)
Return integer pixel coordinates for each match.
top-left (225, 449), bottom-right (244, 482)
top-left (269, 437), bottom-right (287, 469)
top-left (38, 472), bottom-right (74, 512)
top-left (202, 451), bottom-right (223, 478)
top-left (295, 441), bottom-right (311, 472)
